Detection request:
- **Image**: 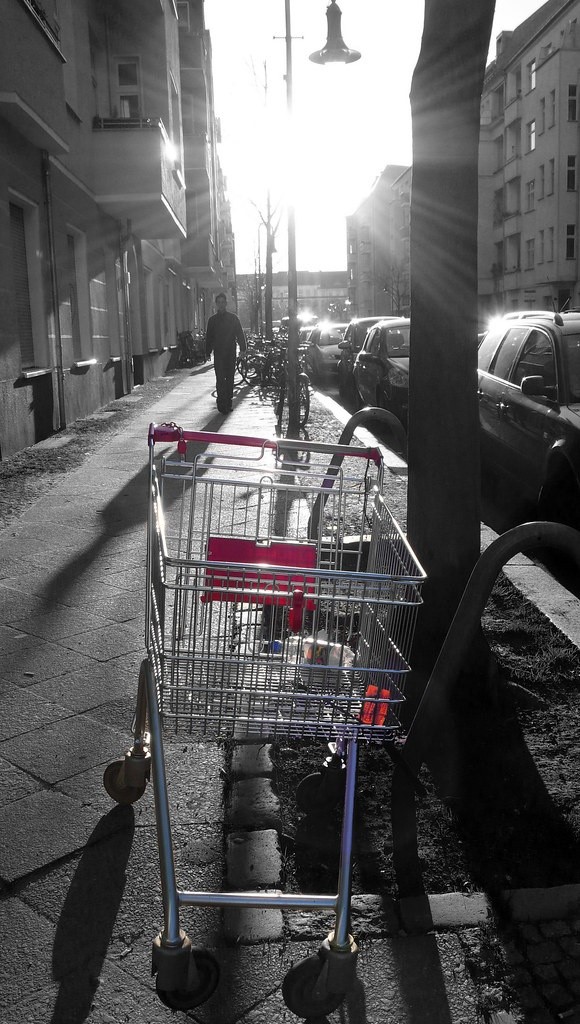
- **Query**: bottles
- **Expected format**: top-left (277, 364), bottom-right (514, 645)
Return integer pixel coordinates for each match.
top-left (270, 635), bottom-right (355, 673)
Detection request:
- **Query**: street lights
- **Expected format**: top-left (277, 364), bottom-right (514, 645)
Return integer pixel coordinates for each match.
top-left (283, 0), bottom-right (362, 431)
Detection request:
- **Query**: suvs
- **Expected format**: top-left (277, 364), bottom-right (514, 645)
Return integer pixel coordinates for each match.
top-left (475, 309), bottom-right (580, 524)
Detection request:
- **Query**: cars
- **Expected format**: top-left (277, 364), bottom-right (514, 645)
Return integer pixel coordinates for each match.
top-left (298, 314), bottom-right (411, 417)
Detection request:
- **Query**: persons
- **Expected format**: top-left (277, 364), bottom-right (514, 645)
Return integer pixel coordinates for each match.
top-left (204, 294), bottom-right (247, 413)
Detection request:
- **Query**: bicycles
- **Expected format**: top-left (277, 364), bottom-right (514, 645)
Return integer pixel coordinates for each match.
top-left (237, 335), bottom-right (319, 427)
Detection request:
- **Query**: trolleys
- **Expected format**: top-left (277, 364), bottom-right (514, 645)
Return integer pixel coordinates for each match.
top-left (100, 419), bottom-right (427, 1017)
